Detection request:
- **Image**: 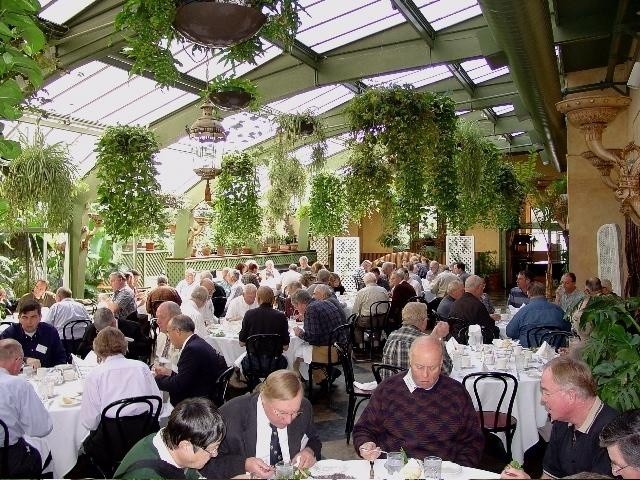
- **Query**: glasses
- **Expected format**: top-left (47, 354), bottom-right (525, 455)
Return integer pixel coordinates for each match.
top-left (414, 363), bottom-right (441, 370)
top-left (270, 403), bottom-right (303, 418)
top-left (541, 389), bottom-right (566, 396)
top-left (194, 445), bottom-right (219, 454)
top-left (610, 461), bottom-right (631, 471)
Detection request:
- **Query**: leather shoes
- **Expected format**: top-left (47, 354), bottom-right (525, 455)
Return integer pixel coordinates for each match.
top-left (320, 366), bottom-right (342, 399)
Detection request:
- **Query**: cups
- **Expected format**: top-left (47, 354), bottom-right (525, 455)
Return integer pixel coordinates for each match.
top-left (386, 452), bottom-right (403, 475)
top-left (24, 365), bottom-right (34, 382)
top-left (207, 316), bottom-right (239, 336)
top-left (422, 456), bottom-right (443, 480)
top-left (274, 460), bottom-right (293, 480)
top-left (568, 336), bottom-right (580, 353)
top-left (36, 367), bottom-right (47, 380)
top-left (494, 307), bottom-right (513, 326)
top-left (449, 335), bottom-right (556, 371)
top-left (286, 316), bottom-right (298, 336)
top-left (47, 379), bottom-right (56, 399)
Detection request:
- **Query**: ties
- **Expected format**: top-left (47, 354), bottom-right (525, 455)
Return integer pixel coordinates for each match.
top-left (161, 334), bottom-right (170, 358)
top-left (269, 423), bottom-right (284, 467)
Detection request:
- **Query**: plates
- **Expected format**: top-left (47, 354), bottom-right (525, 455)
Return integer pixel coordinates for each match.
top-left (55, 398), bottom-right (81, 408)
top-left (440, 460), bottom-right (463, 476)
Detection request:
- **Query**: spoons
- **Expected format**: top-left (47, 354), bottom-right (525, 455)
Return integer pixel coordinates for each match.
top-left (360, 448), bottom-right (403, 460)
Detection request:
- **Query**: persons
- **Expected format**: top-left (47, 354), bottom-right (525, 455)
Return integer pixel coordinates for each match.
top-left (0, 255), bottom-right (640, 480)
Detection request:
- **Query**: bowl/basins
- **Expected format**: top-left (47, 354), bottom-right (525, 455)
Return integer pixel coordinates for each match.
top-left (315, 459), bottom-right (342, 472)
